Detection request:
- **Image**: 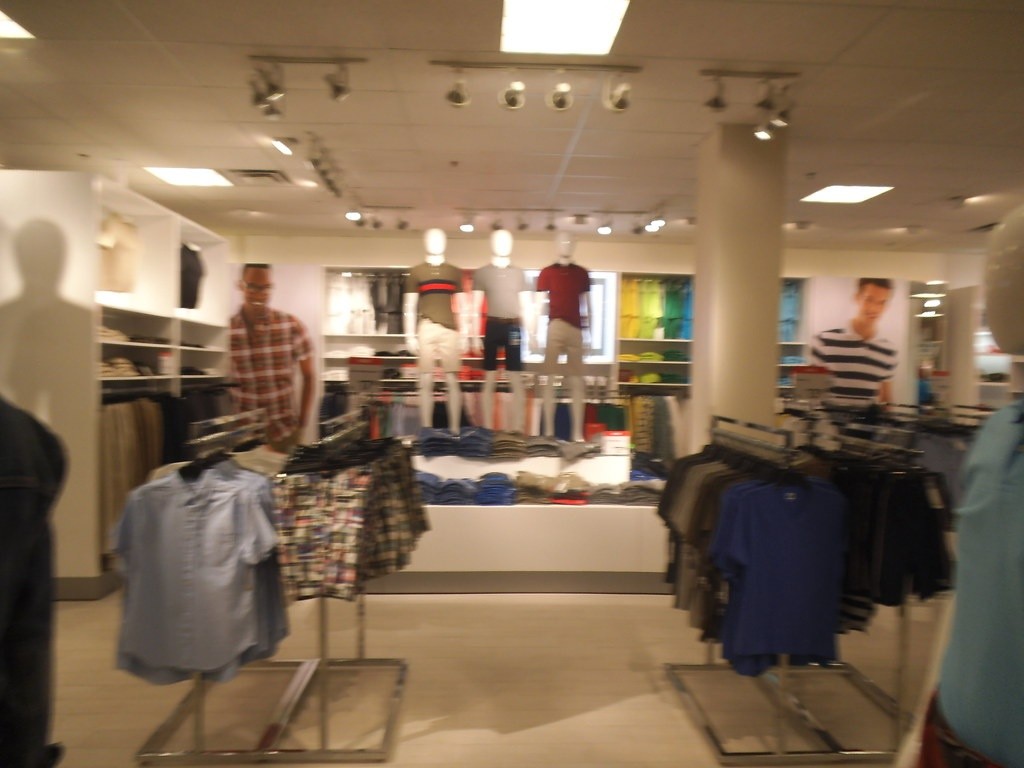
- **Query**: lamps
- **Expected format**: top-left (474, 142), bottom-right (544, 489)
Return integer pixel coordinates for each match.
top-left (257, 67), bottom-right (286, 101)
top-left (701, 76), bottom-right (727, 112)
top-left (547, 71), bottom-right (574, 111)
top-left (546, 215), bottom-right (557, 231)
top-left (445, 72), bottom-right (472, 108)
top-left (460, 216), bottom-right (475, 233)
top-left (754, 82), bottom-right (776, 112)
top-left (263, 104), bottom-right (282, 121)
top-left (306, 137), bottom-right (343, 198)
top-left (371, 215), bottom-right (383, 228)
top-left (489, 216), bottom-right (504, 231)
top-left (752, 124), bottom-right (778, 141)
top-left (770, 103), bottom-right (796, 128)
top-left (325, 66), bottom-right (352, 102)
top-left (515, 215), bottom-right (531, 231)
top-left (396, 214), bottom-right (409, 230)
top-left (249, 78), bottom-right (271, 109)
top-left (630, 218), bottom-right (644, 234)
top-left (496, 71), bottom-right (526, 110)
top-left (602, 70), bottom-right (629, 111)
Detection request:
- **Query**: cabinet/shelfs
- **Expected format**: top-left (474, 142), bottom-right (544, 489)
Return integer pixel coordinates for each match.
top-left (0, 169), bottom-right (225, 601)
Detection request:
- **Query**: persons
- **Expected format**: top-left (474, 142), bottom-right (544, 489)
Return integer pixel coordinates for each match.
top-left (808, 278), bottom-right (898, 403)
top-left (470, 229), bottom-right (532, 432)
top-left (401, 229), bottom-right (467, 433)
top-left (897, 203), bottom-right (1024, 768)
top-left (532, 231), bottom-right (594, 442)
top-left (231, 264), bottom-right (318, 454)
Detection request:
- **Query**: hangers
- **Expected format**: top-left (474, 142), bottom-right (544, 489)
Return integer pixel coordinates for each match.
top-left (278, 407), bottom-right (398, 477)
top-left (703, 403), bottom-right (986, 498)
top-left (174, 422), bottom-right (271, 482)
